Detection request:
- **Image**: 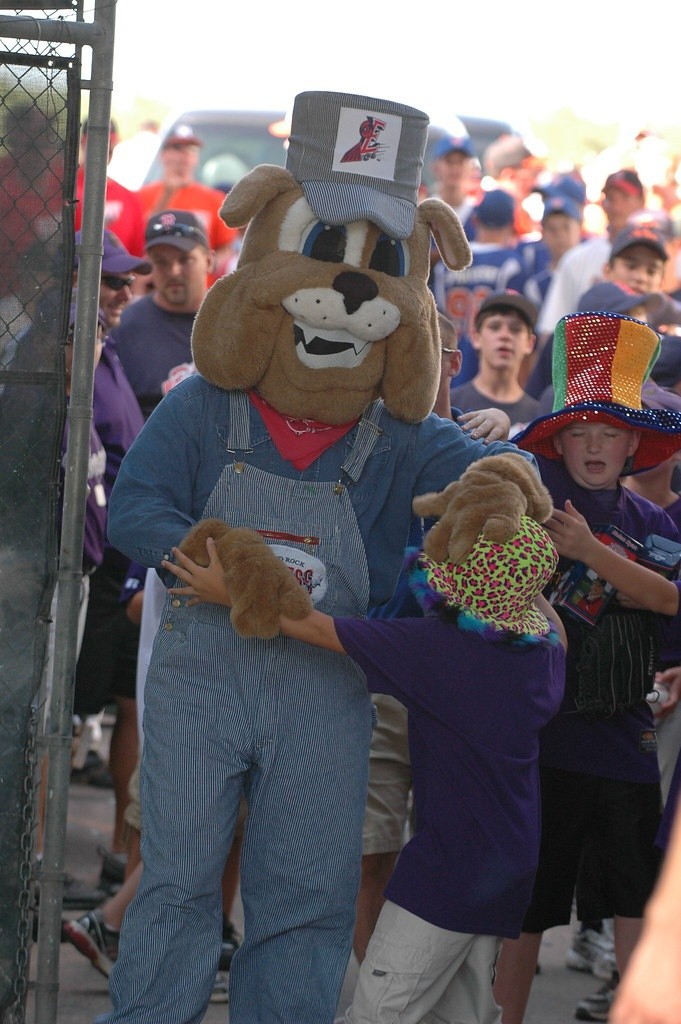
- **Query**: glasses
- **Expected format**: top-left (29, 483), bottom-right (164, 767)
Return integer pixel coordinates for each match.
top-left (101, 275), bottom-right (135, 290)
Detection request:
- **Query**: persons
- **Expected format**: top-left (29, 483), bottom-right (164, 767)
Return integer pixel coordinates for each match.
top-left (0, 105), bottom-right (681, 1024)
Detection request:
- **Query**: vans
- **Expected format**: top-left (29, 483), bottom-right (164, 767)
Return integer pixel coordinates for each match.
top-left (142, 105), bottom-right (537, 209)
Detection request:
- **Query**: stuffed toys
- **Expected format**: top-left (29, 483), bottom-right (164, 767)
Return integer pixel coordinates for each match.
top-left (92, 90), bottom-right (554, 1024)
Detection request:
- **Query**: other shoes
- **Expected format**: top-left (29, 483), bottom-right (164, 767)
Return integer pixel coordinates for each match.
top-left (68, 751), bottom-right (113, 790)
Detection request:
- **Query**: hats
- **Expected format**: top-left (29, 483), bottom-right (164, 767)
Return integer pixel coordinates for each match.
top-left (144, 210), bottom-right (209, 253)
top-left (417, 514), bottom-right (558, 635)
top-left (531, 173), bottom-right (586, 203)
top-left (161, 125), bottom-right (201, 146)
top-left (602, 170), bottom-right (643, 198)
top-left (432, 135), bottom-right (473, 159)
top-left (609, 224), bottom-right (667, 261)
top-left (473, 190), bottom-right (515, 227)
top-left (483, 134), bottom-right (531, 179)
top-left (541, 194), bottom-right (580, 226)
top-left (74, 228), bottom-right (152, 275)
top-left (52, 300), bottom-right (108, 338)
top-left (508, 312), bottom-right (681, 477)
top-left (81, 118), bottom-right (117, 144)
top-left (578, 281), bottom-right (663, 317)
top-left (473, 287), bottom-right (538, 327)
top-left (652, 335), bottom-right (681, 388)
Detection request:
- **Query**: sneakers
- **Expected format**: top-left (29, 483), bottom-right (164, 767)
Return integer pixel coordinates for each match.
top-left (566, 927), bottom-right (616, 979)
top-left (97, 845), bottom-right (128, 898)
top-left (574, 969), bottom-right (620, 1022)
top-left (62, 909), bottom-right (120, 979)
top-left (210, 939), bottom-right (240, 1003)
